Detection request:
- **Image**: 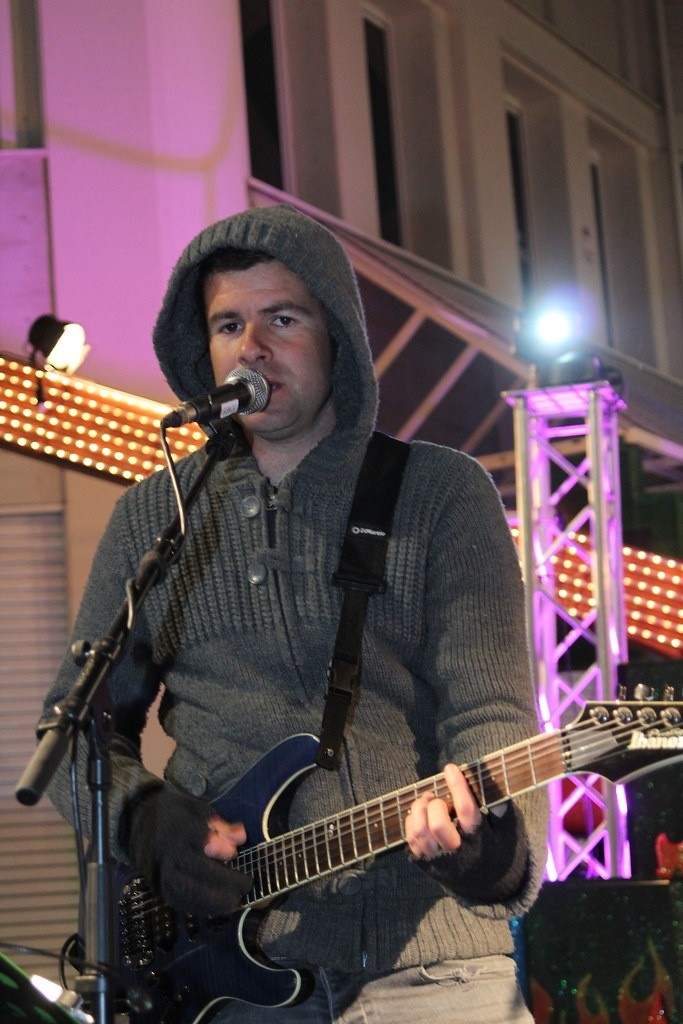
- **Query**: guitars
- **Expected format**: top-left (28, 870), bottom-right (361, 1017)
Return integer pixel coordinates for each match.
top-left (76, 681), bottom-right (683, 1024)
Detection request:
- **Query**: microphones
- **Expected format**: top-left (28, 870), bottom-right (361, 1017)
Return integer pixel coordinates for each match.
top-left (162, 368), bottom-right (271, 428)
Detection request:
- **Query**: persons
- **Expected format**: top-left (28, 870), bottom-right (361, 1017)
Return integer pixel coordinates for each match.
top-left (36, 203), bottom-right (550, 1024)
